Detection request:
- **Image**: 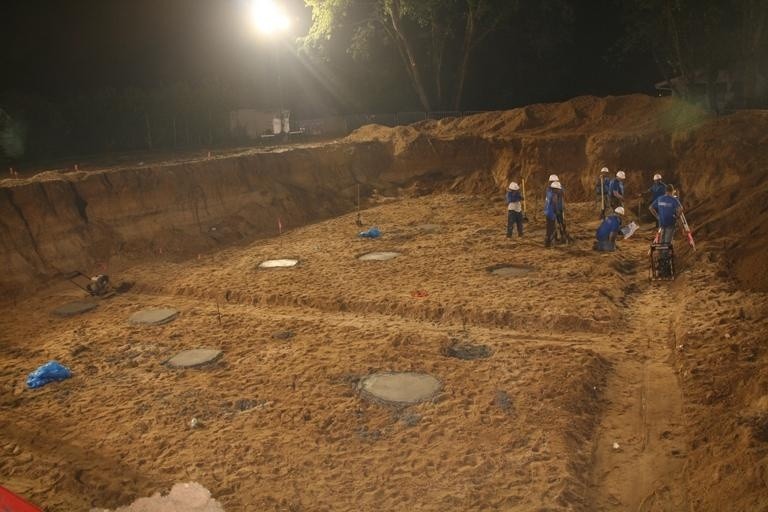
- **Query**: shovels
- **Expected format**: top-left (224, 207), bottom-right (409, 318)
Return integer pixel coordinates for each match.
top-left (355, 184), bottom-right (361, 226)
top-left (522, 178), bottom-right (529, 222)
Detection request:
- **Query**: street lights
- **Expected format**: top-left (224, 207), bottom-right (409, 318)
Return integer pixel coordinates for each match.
top-left (256, 2), bottom-right (293, 141)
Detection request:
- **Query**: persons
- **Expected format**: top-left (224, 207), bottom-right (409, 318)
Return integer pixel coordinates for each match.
top-left (637, 173), bottom-right (665, 228)
top-left (648, 181), bottom-right (684, 245)
top-left (542, 181), bottom-right (562, 248)
top-left (506, 181), bottom-right (524, 243)
top-left (606, 170), bottom-right (626, 215)
top-left (548, 174), bottom-right (570, 244)
top-left (590, 206), bottom-right (624, 252)
top-left (593, 167), bottom-right (610, 217)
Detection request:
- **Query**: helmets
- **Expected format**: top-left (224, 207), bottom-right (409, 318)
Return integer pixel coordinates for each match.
top-left (616, 171), bottom-right (625, 178)
top-left (549, 175), bottom-right (561, 189)
top-left (654, 174), bottom-right (661, 180)
top-left (615, 207), bottom-right (624, 215)
top-left (509, 182), bottom-right (520, 190)
top-left (601, 168), bottom-right (608, 173)
